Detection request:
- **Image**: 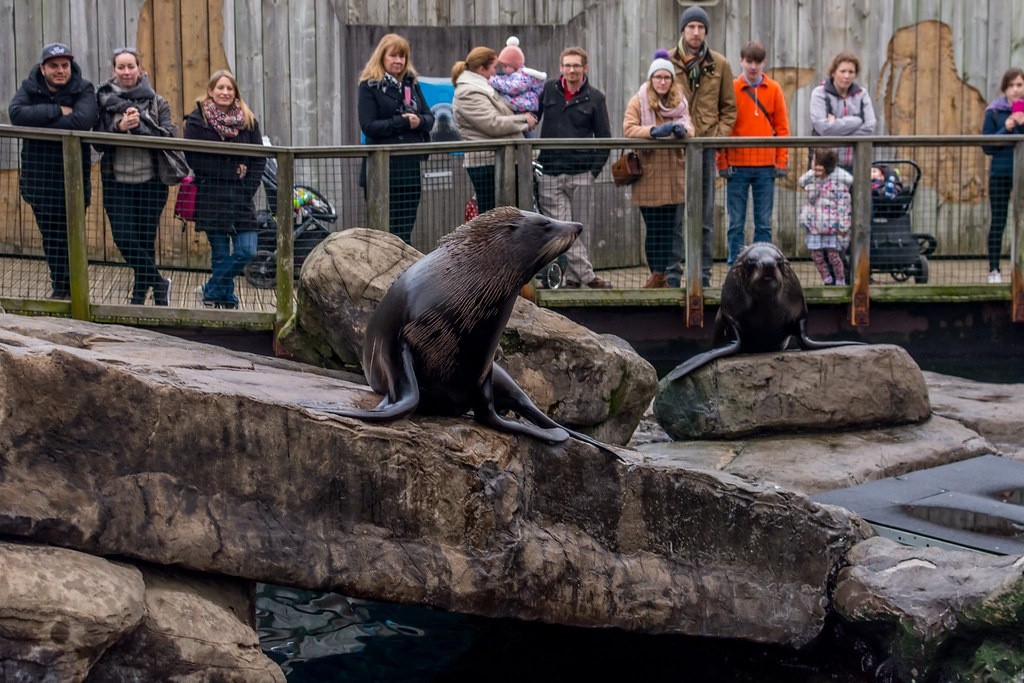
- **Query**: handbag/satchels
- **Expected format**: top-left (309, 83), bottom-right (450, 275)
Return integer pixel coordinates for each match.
top-left (155, 145), bottom-right (190, 186)
top-left (175, 176), bottom-right (198, 221)
top-left (611, 152), bottom-right (643, 187)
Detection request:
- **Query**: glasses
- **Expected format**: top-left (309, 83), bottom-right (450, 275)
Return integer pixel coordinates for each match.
top-left (653, 76), bottom-right (671, 82)
top-left (562, 64), bottom-right (583, 69)
top-left (114, 47), bottom-right (136, 54)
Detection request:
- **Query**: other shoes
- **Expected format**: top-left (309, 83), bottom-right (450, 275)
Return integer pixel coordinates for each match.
top-left (987, 269), bottom-right (1001, 283)
top-left (644, 273), bottom-right (663, 287)
top-left (565, 281), bottom-right (579, 289)
top-left (588, 278), bottom-right (612, 289)
top-left (663, 279), bottom-right (672, 287)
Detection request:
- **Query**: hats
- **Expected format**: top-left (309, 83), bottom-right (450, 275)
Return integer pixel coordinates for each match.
top-left (497, 36), bottom-right (524, 69)
top-left (679, 5), bottom-right (709, 35)
top-left (41, 43), bottom-right (74, 65)
top-left (647, 50), bottom-right (675, 81)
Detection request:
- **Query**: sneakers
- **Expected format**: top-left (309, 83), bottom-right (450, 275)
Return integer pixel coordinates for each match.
top-left (194, 285), bottom-right (218, 309)
top-left (153, 278), bottom-right (171, 306)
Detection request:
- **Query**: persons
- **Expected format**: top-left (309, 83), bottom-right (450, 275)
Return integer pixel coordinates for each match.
top-left (797, 147), bottom-right (854, 286)
top-left (531, 47), bottom-right (612, 288)
top-left (7, 42), bottom-right (97, 300)
top-left (451, 48), bottom-right (539, 216)
top-left (623, 49), bottom-right (697, 288)
top-left (354, 32), bottom-right (434, 248)
top-left (183, 69), bottom-right (266, 306)
top-left (980, 67), bottom-right (1024, 283)
top-left (869, 168), bottom-right (903, 195)
top-left (91, 47), bottom-right (179, 307)
top-left (486, 36), bottom-right (546, 140)
top-left (664, 6), bottom-right (739, 289)
top-left (715, 41), bottom-right (790, 268)
top-left (808, 52), bottom-right (876, 286)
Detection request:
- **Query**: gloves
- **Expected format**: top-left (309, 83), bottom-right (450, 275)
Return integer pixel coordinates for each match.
top-left (773, 169), bottom-right (786, 177)
top-left (719, 169), bottom-right (730, 177)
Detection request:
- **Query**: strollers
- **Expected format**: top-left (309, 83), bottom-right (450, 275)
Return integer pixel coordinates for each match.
top-left (238, 136), bottom-right (338, 288)
top-left (830, 160), bottom-right (936, 284)
